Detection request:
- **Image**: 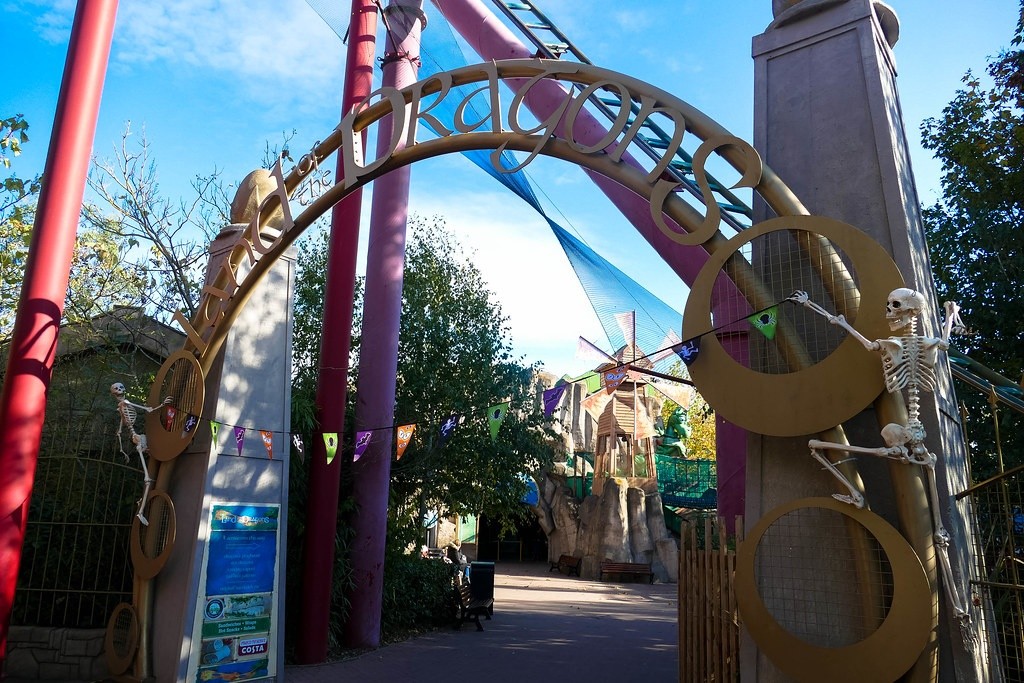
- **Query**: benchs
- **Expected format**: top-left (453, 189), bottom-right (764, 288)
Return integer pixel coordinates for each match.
top-left (451, 581), bottom-right (494, 632)
top-left (599, 561), bottom-right (655, 584)
top-left (548, 553), bottom-right (583, 575)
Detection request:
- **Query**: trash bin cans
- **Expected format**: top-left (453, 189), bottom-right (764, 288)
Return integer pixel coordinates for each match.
top-left (468, 562), bottom-right (495, 616)
